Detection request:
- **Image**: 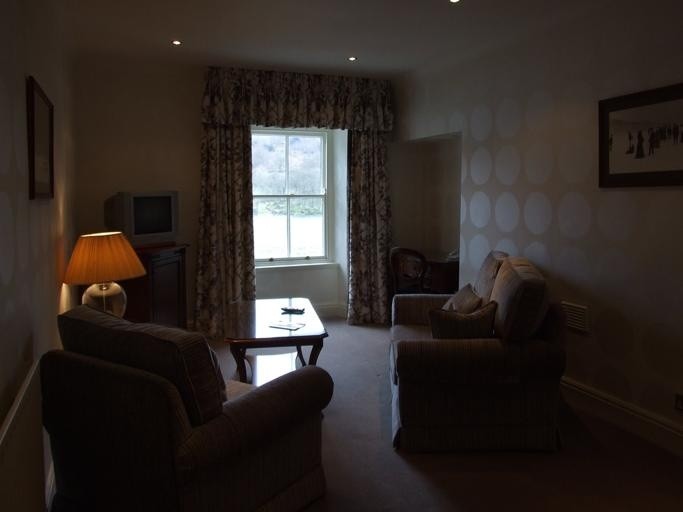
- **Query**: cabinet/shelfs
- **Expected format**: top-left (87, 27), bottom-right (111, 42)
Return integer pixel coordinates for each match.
top-left (117, 244), bottom-right (191, 331)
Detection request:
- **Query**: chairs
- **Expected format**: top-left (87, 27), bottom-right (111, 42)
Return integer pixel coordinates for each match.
top-left (389, 251), bottom-right (564, 451)
top-left (40, 304), bottom-right (334, 510)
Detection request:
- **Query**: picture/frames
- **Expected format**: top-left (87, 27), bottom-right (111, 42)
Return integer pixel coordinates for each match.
top-left (598, 83), bottom-right (683, 187)
top-left (28, 75), bottom-right (55, 200)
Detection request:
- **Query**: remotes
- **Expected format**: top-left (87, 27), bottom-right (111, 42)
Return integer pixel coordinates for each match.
top-left (282, 305), bottom-right (305, 314)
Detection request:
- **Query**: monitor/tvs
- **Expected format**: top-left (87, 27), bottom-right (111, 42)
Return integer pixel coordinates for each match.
top-left (103, 188), bottom-right (181, 248)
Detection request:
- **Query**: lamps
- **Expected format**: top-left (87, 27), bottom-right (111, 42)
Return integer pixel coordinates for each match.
top-left (62, 230), bottom-right (147, 320)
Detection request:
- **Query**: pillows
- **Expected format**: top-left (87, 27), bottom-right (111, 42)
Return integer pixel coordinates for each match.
top-left (428, 283), bottom-right (497, 339)
